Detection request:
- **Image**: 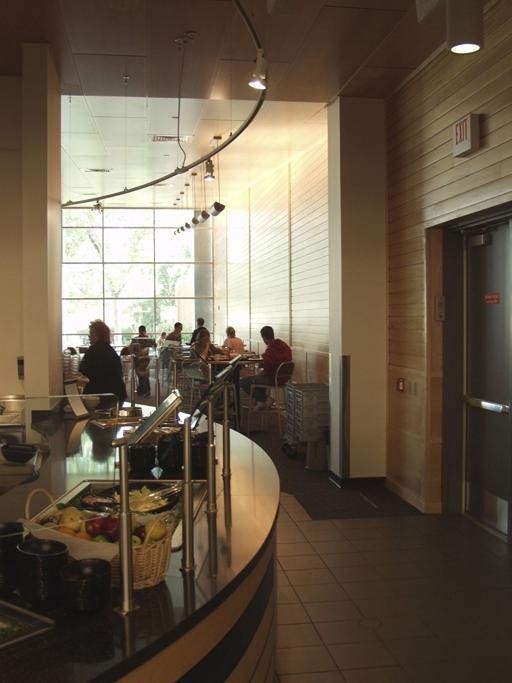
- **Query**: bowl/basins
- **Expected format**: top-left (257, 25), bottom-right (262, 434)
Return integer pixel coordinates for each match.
top-left (82, 396), bottom-right (101, 409)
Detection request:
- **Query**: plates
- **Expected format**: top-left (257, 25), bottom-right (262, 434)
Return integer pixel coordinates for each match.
top-left (80, 480), bottom-right (179, 515)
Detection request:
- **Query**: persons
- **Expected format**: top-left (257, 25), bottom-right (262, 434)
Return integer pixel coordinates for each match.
top-left (131, 318), bottom-right (292, 413)
top-left (79, 318), bottom-right (128, 401)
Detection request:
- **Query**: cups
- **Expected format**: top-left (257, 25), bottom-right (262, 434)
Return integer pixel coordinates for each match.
top-left (0, 519), bottom-right (28, 594)
top-left (128, 429), bottom-right (214, 480)
top-left (63, 556), bottom-right (114, 611)
top-left (15, 537), bottom-right (69, 606)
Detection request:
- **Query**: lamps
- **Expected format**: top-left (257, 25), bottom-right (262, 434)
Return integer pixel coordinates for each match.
top-left (248, 47), bottom-right (269, 92)
top-left (172, 135), bottom-right (226, 238)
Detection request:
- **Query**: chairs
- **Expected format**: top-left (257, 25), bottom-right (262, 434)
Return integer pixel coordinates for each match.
top-left (68, 341), bottom-right (295, 442)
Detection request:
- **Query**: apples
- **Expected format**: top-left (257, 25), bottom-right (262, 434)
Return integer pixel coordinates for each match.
top-left (40, 507), bottom-right (174, 547)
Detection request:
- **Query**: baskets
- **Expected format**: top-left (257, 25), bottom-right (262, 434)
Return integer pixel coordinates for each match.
top-left (25, 488), bottom-right (176, 590)
